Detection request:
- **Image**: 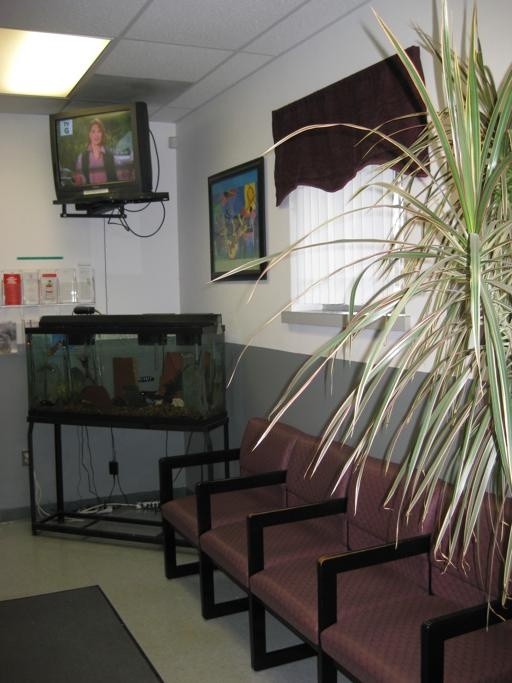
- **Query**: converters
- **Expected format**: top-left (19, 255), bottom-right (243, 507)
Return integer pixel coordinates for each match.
top-left (109, 462), bottom-right (118, 474)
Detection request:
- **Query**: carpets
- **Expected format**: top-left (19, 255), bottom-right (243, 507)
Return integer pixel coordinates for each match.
top-left (1, 585), bottom-right (166, 682)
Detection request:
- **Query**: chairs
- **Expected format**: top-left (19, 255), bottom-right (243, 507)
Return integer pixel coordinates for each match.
top-left (309, 477), bottom-right (512, 683)
top-left (160, 421), bottom-right (298, 621)
top-left (196, 431), bottom-right (356, 671)
top-left (241, 454), bottom-right (426, 683)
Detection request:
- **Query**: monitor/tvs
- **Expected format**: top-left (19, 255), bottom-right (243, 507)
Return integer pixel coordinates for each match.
top-left (48, 101), bottom-right (153, 201)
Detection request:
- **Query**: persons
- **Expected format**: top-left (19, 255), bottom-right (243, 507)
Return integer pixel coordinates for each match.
top-left (73, 117), bottom-right (135, 184)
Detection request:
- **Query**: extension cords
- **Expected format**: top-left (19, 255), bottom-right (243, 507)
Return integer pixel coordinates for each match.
top-left (77, 504), bottom-right (113, 514)
top-left (136, 500), bottom-right (160, 510)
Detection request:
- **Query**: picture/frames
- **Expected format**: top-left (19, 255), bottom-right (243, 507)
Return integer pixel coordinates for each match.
top-left (207, 156), bottom-right (267, 280)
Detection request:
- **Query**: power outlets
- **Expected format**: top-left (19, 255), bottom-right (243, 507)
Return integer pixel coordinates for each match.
top-left (21, 449), bottom-right (32, 469)
